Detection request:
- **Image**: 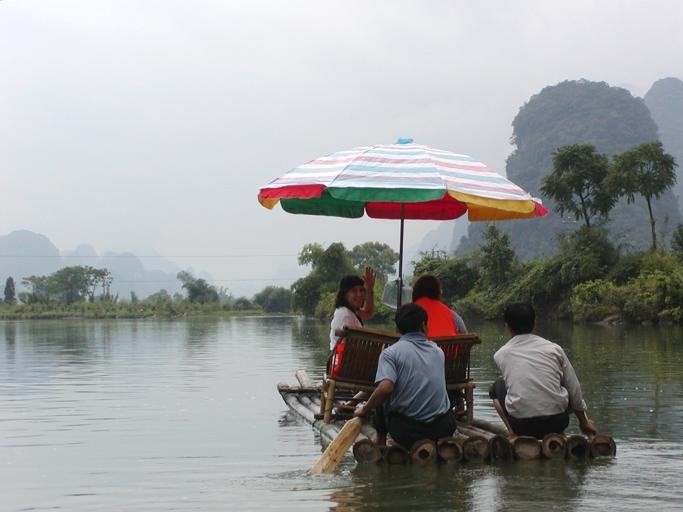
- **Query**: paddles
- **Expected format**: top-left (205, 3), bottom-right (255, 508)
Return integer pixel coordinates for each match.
top-left (309, 405), bottom-right (374, 475)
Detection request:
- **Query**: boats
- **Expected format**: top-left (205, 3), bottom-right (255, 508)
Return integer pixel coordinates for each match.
top-left (277, 326), bottom-right (617, 463)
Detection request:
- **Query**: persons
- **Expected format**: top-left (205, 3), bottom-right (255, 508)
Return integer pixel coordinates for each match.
top-left (489, 302), bottom-right (597, 441)
top-left (329, 266), bottom-right (377, 412)
top-left (412, 274), bottom-right (468, 360)
top-left (353, 303), bottom-right (457, 447)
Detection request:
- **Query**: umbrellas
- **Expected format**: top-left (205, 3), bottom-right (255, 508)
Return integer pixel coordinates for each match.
top-left (257, 138), bottom-right (549, 332)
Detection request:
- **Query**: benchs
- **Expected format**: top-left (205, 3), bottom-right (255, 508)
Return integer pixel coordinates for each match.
top-left (320, 325), bottom-right (482, 423)
